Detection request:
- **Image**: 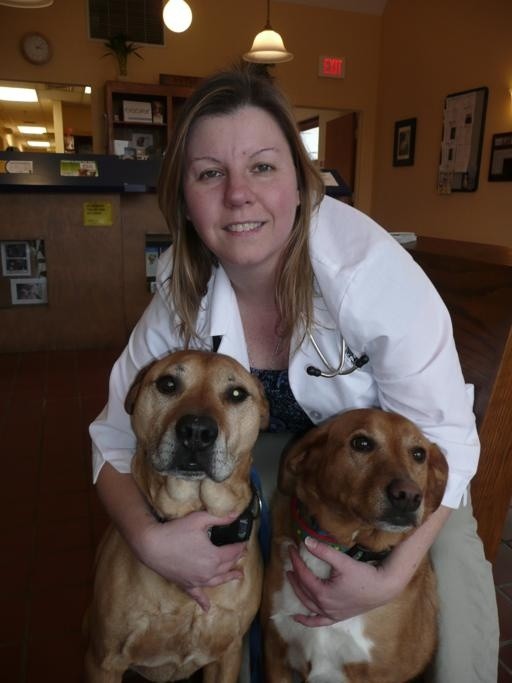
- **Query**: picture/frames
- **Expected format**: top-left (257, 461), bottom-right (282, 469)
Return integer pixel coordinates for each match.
top-left (437, 86), bottom-right (489, 191)
top-left (486, 131), bottom-right (512, 182)
top-left (392, 117), bottom-right (416, 166)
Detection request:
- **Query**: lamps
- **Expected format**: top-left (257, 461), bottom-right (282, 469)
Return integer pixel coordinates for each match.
top-left (242, 0), bottom-right (293, 64)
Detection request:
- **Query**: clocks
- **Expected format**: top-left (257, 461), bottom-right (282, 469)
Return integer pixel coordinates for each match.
top-left (22, 31), bottom-right (51, 65)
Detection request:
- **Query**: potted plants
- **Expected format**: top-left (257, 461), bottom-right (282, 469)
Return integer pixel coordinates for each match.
top-left (105, 35), bottom-right (145, 83)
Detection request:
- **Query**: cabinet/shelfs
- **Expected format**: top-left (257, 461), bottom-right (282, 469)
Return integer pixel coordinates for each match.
top-left (102, 83), bottom-right (171, 160)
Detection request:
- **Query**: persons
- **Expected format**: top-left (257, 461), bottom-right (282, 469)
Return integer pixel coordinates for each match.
top-left (86, 63), bottom-right (503, 682)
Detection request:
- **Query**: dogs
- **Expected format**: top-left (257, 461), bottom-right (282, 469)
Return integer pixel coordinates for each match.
top-left (260, 407), bottom-right (448, 683)
top-left (79, 346), bottom-right (271, 683)
